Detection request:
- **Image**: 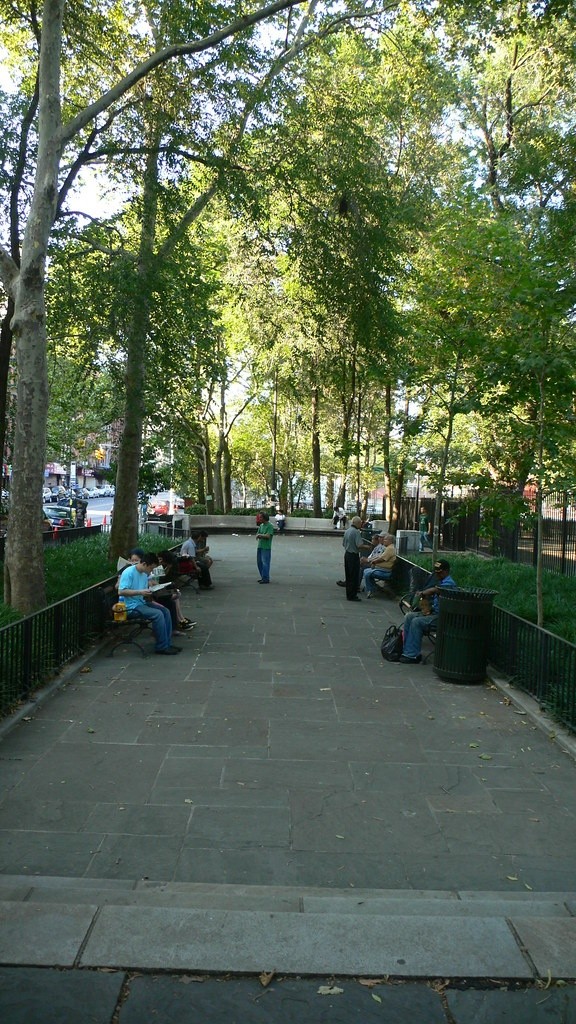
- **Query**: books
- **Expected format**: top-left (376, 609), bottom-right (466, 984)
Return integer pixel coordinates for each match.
top-left (148, 582), bottom-right (171, 592)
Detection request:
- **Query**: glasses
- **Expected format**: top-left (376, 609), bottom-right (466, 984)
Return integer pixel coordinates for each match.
top-left (379, 536), bottom-right (383, 538)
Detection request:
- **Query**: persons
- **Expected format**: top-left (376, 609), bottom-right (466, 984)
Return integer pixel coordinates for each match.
top-left (180, 530), bottom-right (216, 590)
top-left (274, 509), bottom-right (287, 536)
top-left (139, 496), bottom-right (148, 522)
top-left (255, 509), bottom-right (274, 584)
top-left (118, 552), bottom-right (184, 655)
top-left (415, 506), bottom-right (435, 552)
top-left (73, 500), bottom-right (87, 528)
top-left (399, 560), bottom-right (457, 664)
top-left (342, 515), bottom-right (372, 602)
top-left (115, 548), bottom-right (197, 636)
top-left (330, 500), bottom-right (397, 602)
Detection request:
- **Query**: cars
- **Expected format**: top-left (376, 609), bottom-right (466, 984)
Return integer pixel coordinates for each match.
top-left (42, 505), bottom-right (75, 530)
top-left (63, 484), bottom-right (116, 499)
top-left (1, 487), bottom-right (10, 513)
top-left (147, 491), bottom-right (185, 516)
top-left (42, 487), bottom-right (53, 503)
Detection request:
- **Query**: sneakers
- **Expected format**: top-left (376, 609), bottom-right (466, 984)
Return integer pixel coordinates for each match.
top-left (184, 618), bottom-right (197, 626)
top-left (176, 621), bottom-right (193, 631)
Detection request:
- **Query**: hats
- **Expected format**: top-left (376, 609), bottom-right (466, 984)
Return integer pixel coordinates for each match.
top-left (432, 559), bottom-right (449, 573)
top-left (377, 531), bottom-right (388, 537)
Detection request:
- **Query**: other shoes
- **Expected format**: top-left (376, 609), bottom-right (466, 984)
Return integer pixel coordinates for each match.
top-left (366, 591), bottom-right (376, 598)
top-left (336, 580), bottom-right (347, 587)
top-left (169, 646), bottom-right (183, 652)
top-left (200, 585), bottom-right (213, 590)
top-left (260, 580), bottom-right (269, 583)
top-left (347, 596), bottom-right (362, 601)
top-left (155, 648), bottom-right (178, 655)
top-left (399, 654), bottom-right (422, 664)
top-left (258, 579), bottom-right (262, 582)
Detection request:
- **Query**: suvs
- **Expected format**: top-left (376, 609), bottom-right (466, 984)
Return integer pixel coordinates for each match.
top-left (47, 486), bottom-right (67, 502)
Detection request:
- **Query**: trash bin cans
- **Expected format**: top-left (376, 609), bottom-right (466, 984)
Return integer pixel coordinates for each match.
top-left (434, 584), bottom-right (499, 685)
top-left (359, 528), bottom-right (383, 558)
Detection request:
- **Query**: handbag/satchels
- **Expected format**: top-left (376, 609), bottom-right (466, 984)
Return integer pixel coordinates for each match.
top-left (381, 625), bottom-right (403, 662)
top-left (152, 591), bottom-right (172, 601)
top-left (421, 596), bottom-right (433, 615)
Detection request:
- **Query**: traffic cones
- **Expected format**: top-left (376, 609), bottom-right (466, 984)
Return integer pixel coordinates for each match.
top-left (102, 515), bottom-right (106, 525)
top-left (87, 518), bottom-right (92, 528)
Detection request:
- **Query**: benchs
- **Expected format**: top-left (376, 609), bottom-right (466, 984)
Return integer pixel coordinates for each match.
top-left (367, 559), bottom-right (397, 599)
top-left (175, 553), bottom-right (202, 594)
top-left (189, 515), bottom-right (347, 535)
top-left (398, 593), bottom-right (439, 664)
top-left (104, 584), bottom-right (155, 656)
